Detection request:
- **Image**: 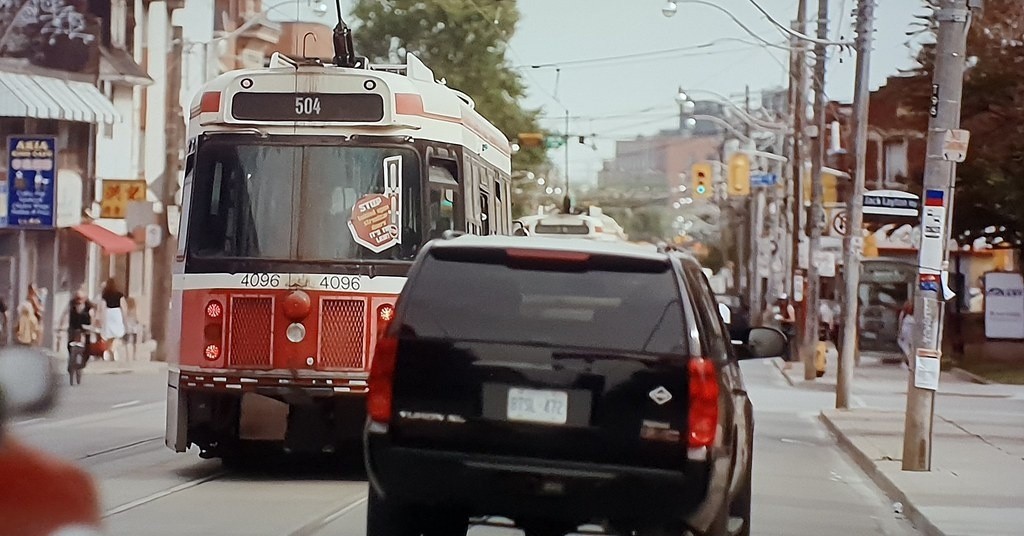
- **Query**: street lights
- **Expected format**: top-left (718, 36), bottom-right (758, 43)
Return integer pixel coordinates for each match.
top-left (201, 0), bottom-right (327, 83)
top-left (663, 0), bottom-right (806, 284)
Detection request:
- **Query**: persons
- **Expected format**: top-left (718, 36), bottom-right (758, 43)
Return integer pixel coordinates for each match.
top-left (819, 300), bottom-right (841, 348)
top-left (0, 282), bottom-right (42, 349)
top-left (854, 294), bottom-right (863, 367)
top-left (68, 278), bottom-right (139, 358)
top-left (775, 293), bottom-right (795, 361)
top-left (899, 301), bottom-right (914, 366)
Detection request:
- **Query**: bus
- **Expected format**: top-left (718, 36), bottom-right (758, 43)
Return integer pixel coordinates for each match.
top-left (165, 51), bottom-right (518, 467)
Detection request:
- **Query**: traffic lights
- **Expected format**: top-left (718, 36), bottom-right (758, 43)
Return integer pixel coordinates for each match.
top-left (691, 162), bottom-right (712, 199)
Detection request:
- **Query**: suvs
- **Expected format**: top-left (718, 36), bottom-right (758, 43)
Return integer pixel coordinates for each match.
top-left (827, 269), bottom-right (914, 363)
top-left (363, 232), bottom-right (754, 536)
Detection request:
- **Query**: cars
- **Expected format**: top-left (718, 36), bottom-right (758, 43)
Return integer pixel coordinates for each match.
top-left (0, 347), bottom-right (102, 536)
top-left (714, 293), bottom-right (751, 336)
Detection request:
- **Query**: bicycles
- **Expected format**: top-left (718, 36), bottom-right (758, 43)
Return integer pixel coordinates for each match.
top-left (54, 326), bottom-right (101, 386)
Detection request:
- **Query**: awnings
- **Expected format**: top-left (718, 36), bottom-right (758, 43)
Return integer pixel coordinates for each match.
top-left (69, 221), bottom-right (137, 254)
top-left (0, 71), bottom-right (123, 124)
